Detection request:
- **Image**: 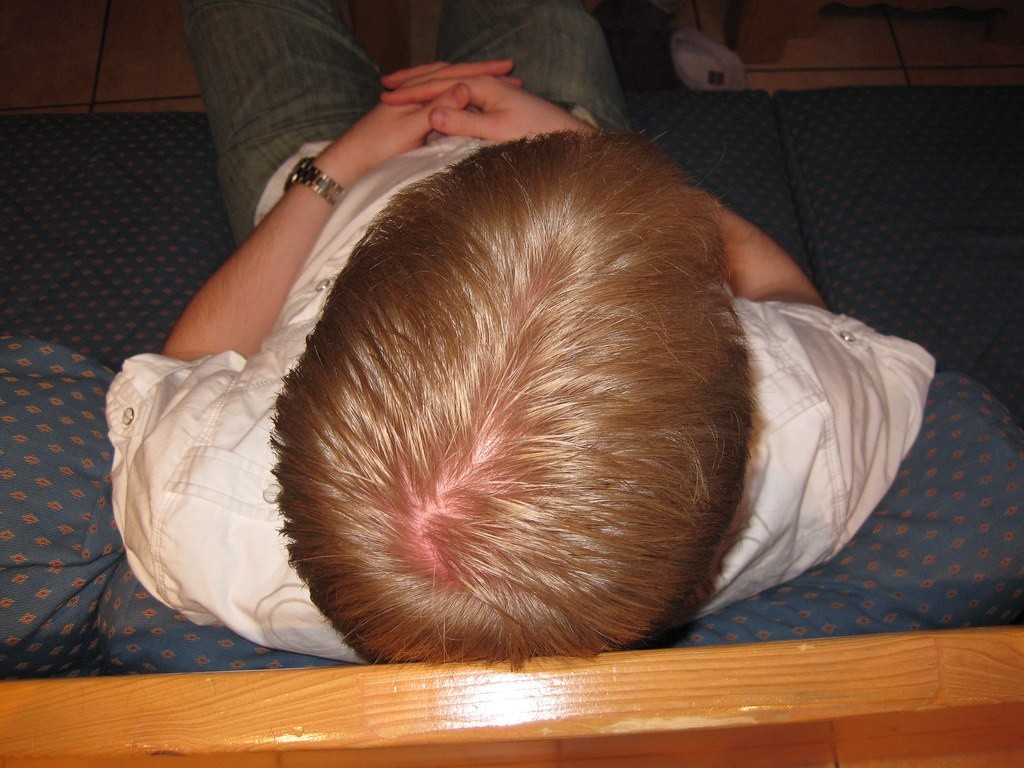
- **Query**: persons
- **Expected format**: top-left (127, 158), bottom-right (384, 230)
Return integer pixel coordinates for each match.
top-left (96, 0), bottom-right (940, 669)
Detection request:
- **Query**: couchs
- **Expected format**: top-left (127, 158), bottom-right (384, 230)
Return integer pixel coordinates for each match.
top-left (0, 81), bottom-right (1023, 767)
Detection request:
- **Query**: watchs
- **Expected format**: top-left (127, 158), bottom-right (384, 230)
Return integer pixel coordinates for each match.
top-left (283, 157), bottom-right (349, 208)
